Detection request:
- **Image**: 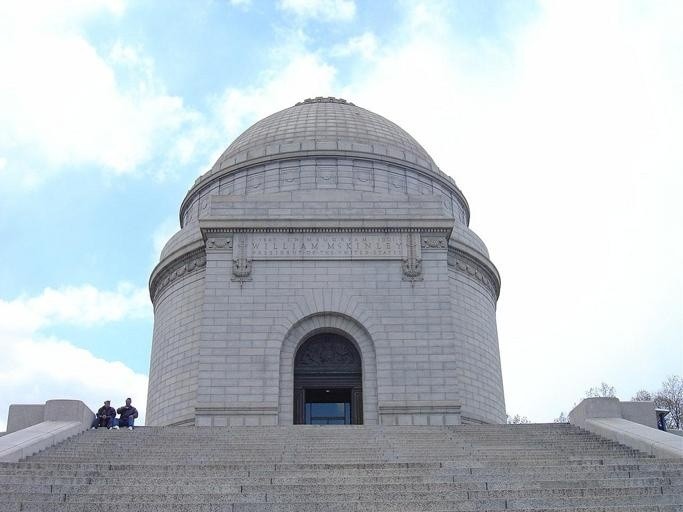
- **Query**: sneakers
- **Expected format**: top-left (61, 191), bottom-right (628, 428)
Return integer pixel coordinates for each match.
top-left (109, 425), bottom-right (133, 431)
top-left (91, 427), bottom-right (97, 430)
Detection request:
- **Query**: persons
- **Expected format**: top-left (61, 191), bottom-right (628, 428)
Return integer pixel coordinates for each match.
top-left (113, 398), bottom-right (138, 430)
top-left (657, 413), bottom-right (667, 430)
top-left (91, 401), bottom-right (116, 430)
